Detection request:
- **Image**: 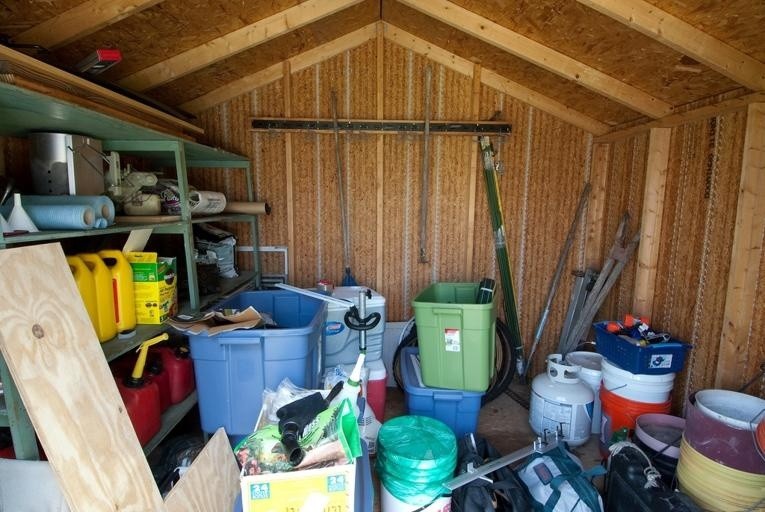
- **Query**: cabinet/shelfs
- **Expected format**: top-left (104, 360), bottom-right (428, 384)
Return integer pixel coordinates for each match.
top-left (0, 81), bottom-right (263, 459)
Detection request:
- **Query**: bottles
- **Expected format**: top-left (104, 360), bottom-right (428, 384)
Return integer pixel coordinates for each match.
top-left (341, 264), bottom-right (356, 287)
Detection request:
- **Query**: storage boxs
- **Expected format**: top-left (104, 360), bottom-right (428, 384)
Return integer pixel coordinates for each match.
top-left (183, 281), bottom-right (499, 512)
top-left (593, 319), bottom-right (693, 374)
top-left (122, 228), bottom-right (178, 325)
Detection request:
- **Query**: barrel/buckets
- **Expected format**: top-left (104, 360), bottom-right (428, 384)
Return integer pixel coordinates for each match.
top-left (77, 253), bottom-right (117, 342)
top-left (135, 355), bottom-right (168, 417)
top-left (66, 256), bottom-right (101, 338)
top-left (98, 248), bottom-right (137, 331)
top-left (564, 350), bottom-right (602, 434)
top-left (674, 391), bottom-right (765, 511)
top-left (631, 414), bottom-right (685, 484)
top-left (158, 343), bottom-right (194, 404)
top-left (375, 416), bottom-right (456, 512)
top-left (112, 372), bottom-right (161, 443)
top-left (600, 361), bottom-right (676, 402)
top-left (600, 381), bottom-right (674, 454)
